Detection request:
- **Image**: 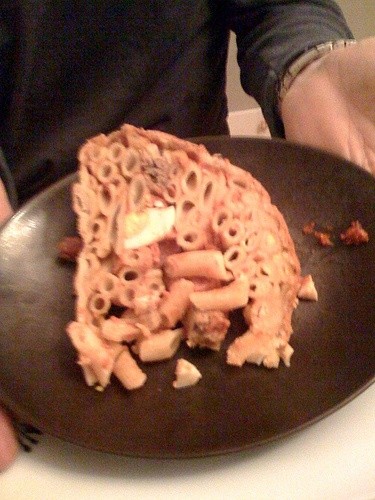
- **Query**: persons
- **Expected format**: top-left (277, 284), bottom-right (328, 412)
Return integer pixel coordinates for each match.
top-left (0, 0), bottom-right (375, 471)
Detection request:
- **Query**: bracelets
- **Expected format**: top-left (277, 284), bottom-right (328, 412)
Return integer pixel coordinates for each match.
top-left (275, 39), bottom-right (359, 118)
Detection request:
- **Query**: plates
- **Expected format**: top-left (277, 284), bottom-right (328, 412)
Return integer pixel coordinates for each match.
top-left (0, 136), bottom-right (375, 459)
